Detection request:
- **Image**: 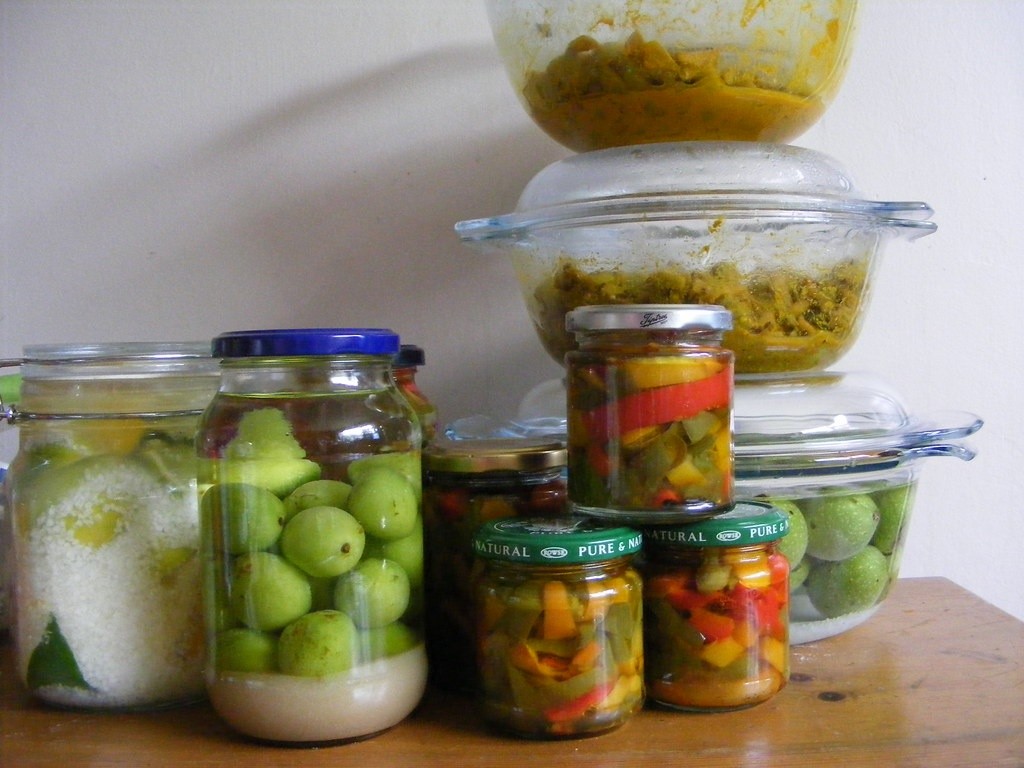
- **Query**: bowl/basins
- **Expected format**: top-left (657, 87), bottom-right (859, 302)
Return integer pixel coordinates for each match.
top-left (447, 377), bottom-right (982, 644)
top-left (454, 142), bottom-right (937, 373)
top-left (484, 0), bottom-right (859, 153)
